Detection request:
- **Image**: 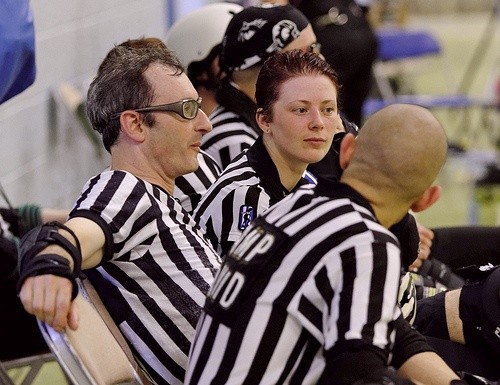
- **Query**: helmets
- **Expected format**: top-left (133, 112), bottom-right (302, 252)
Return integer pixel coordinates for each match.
top-left (166, 3), bottom-right (244, 78)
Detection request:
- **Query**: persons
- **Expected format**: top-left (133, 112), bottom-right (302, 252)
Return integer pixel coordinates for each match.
top-left (0, 0), bottom-right (500, 385)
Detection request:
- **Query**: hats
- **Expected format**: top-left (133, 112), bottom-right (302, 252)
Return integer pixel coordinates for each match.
top-left (221, 4), bottom-right (310, 72)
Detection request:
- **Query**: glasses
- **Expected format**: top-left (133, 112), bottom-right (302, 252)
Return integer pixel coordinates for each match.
top-left (109, 97), bottom-right (203, 119)
top-left (309, 43), bottom-right (321, 57)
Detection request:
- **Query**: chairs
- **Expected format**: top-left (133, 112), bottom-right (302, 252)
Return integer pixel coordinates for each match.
top-left (36, 273), bottom-right (146, 385)
